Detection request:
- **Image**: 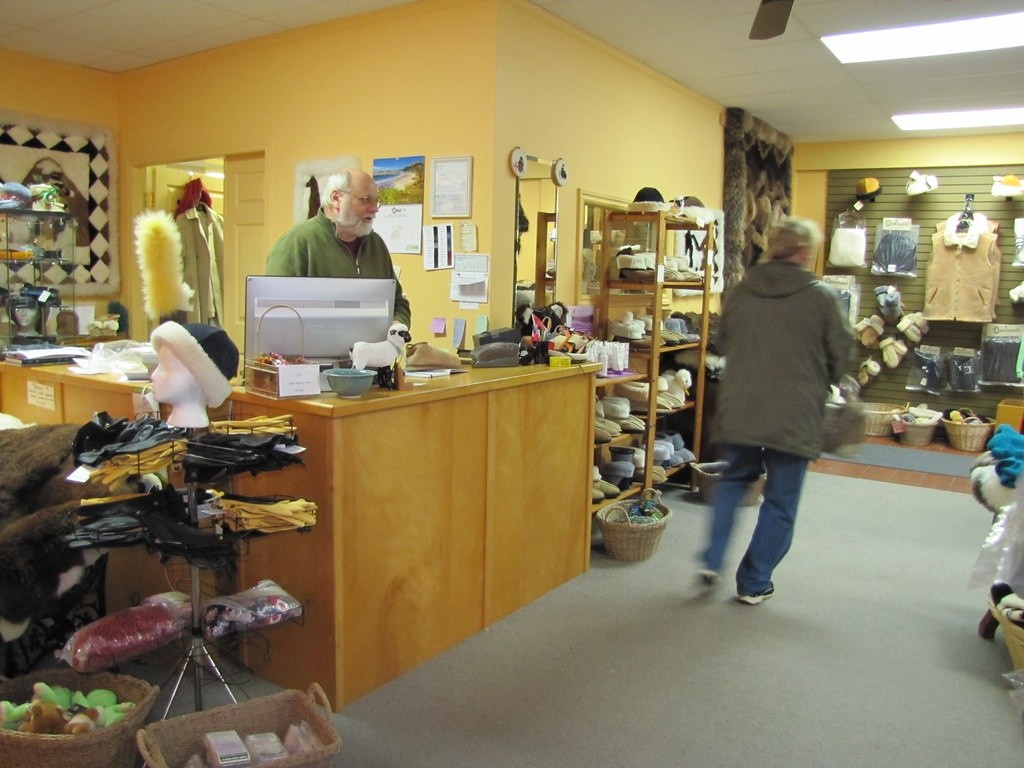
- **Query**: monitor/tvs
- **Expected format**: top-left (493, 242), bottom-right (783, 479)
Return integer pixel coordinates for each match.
top-left (244, 276), bottom-right (397, 377)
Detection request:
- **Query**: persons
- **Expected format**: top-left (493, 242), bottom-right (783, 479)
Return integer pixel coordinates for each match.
top-left (149, 321), bottom-right (239, 428)
top-left (267, 168), bottom-right (412, 333)
top-left (7, 297), bottom-right (50, 337)
top-left (694, 219), bottom-right (851, 603)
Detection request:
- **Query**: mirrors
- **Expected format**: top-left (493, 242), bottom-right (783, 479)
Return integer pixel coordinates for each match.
top-left (511, 148), bottom-right (559, 332)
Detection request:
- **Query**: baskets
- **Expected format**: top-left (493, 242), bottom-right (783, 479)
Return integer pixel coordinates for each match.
top-left (596, 488), bottom-right (671, 560)
top-left (892, 403), bottom-right (944, 445)
top-left (987, 592), bottom-right (1024, 672)
top-left (690, 462), bottom-right (765, 505)
top-left (856, 401), bottom-right (909, 437)
top-left (0, 668), bottom-right (160, 767)
top-left (136, 682), bottom-right (342, 768)
top-left (941, 417), bottom-right (995, 451)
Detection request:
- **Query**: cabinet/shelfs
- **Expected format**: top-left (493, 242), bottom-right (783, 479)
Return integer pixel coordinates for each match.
top-left (534, 212), bottom-right (556, 310)
top-left (0, 208), bottom-right (80, 351)
top-left (591, 210), bottom-right (715, 514)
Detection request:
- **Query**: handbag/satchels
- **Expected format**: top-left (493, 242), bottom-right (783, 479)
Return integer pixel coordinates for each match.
top-left (909, 346), bottom-right (982, 392)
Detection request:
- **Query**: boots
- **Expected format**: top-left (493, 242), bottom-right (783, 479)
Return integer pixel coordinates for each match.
top-left (592, 244), bottom-right (700, 502)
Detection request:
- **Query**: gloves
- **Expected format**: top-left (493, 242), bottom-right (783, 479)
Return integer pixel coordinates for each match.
top-left (852, 285), bottom-right (929, 387)
top-left (58, 409), bottom-right (319, 571)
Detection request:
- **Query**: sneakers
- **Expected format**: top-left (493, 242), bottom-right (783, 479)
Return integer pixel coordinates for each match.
top-left (696, 568), bottom-right (718, 586)
top-left (738, 581), bottom-right (774, 604)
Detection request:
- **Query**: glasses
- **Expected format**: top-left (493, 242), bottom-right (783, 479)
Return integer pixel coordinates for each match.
top-left (340, 190), bottom-right (380, 208)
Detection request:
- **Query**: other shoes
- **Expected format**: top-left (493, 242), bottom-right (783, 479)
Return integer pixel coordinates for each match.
top-left (405, 342), bottom-right (461, 370)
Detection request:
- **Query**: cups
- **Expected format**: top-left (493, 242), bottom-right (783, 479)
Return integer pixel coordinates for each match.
top-left (533, 341), bottom-right (548, 364)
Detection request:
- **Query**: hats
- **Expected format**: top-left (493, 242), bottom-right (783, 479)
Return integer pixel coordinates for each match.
top-left (628, 187), bottom-right (670, 212)
top-left (991, 175), bottom-right (1024, 197)
top-left (906, 172), bottom-right (938, 196)
top-left (856, 178), bottom-right (882, 199)
top-left (150, 320), bottom-right (240, 408)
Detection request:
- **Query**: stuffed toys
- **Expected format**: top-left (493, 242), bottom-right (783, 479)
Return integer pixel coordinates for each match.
top-left (0, 682), bottom-right (137, 735)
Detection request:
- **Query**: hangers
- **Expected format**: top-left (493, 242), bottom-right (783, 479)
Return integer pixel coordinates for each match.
top-left (956, 220), bottom-right (971, 233)
top-left (959, 201), bottom-right (974, 220)
top-left (196, 203), bottom-right (207, 214)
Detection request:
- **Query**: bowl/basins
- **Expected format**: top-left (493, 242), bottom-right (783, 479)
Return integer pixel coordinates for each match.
top-left (322, 368), bottom-right (379, 399)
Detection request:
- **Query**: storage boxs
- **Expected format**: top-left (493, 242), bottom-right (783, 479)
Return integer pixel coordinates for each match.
top-left (244, 358), bottom-right (320, 401)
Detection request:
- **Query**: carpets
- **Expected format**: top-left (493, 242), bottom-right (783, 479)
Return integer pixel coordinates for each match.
top-left (818, 442), bottom-right (977, 478)
